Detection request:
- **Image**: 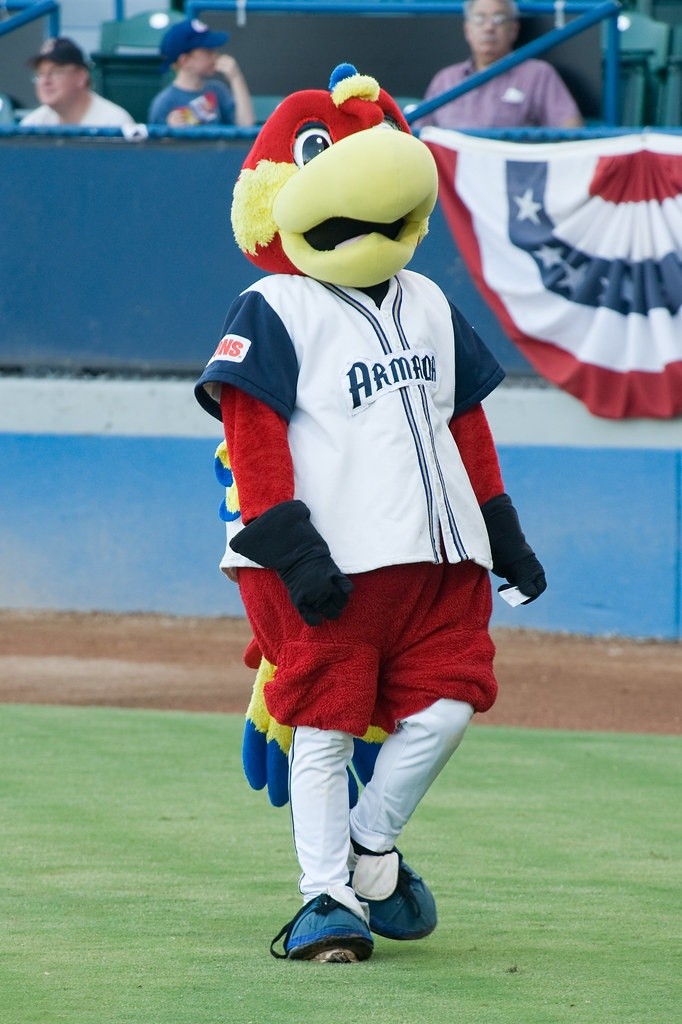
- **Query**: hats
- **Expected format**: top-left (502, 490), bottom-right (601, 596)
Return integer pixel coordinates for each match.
top-left (160, 17), bottom-right (229, 65)
top-left (26, 36), bottom-right (84, 67)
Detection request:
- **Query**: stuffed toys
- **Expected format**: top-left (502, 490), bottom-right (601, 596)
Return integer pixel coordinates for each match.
top-left (193, 61), bottom-right (549, 964)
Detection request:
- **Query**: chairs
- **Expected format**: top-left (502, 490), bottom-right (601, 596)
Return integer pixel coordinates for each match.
top-left (0, 1), bottom-right (682, 131)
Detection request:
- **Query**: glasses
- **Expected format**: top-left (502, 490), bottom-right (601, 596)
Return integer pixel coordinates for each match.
top-left (469, 12), bottom-right (509, 26)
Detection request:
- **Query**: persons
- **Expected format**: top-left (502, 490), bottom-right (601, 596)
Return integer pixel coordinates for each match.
top-left (412, 0), bottom-right (584, 128)
top-left (17, 37), bottom-right (138, 127)
top-left (148, 17), bottom-right (255, 127)
